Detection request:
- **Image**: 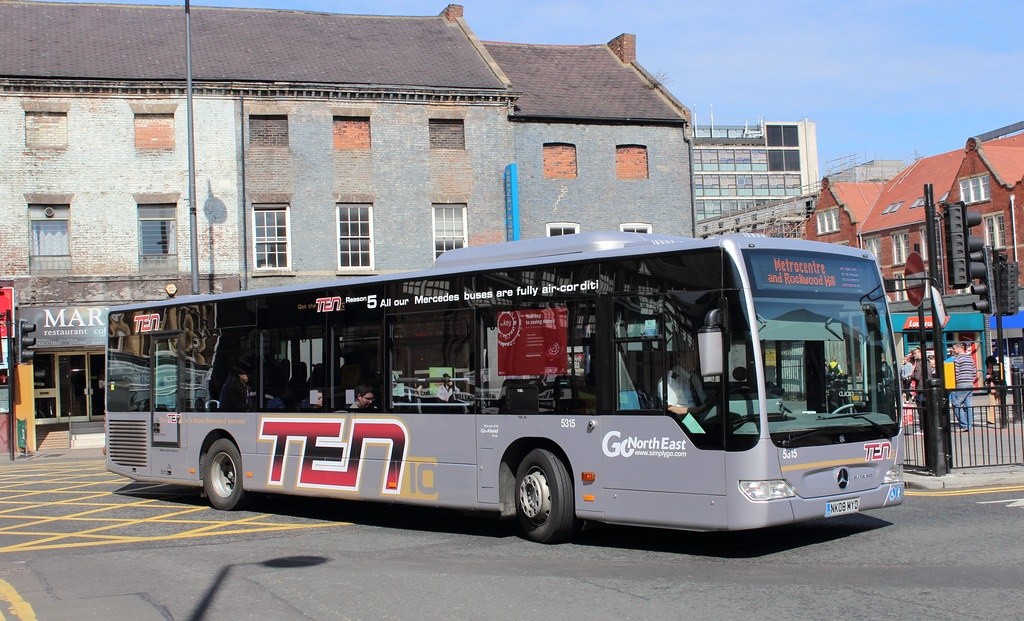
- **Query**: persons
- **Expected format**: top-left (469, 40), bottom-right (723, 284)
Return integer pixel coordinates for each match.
top-left (951, 340), bottom-right (977, 432)
top-left (438, 373), bottom-right (461, 401)
top-left (830, 358), bottom-right (844, 374)
top-left (985, 354), bottom-right (1011, 424)
top-left (348, 384), bottom-right (381, 408)
top-left (902, 342), bottom-right (1024, 407)
top-left (220, 364), bottom-right (259, 410)
top-left (564, 375), bottom-right (596, 415)
top-left (656, 341), bottom-right (746, 434)
top-left (910, 346), bottom-right (936, 436)
top-left (309, 389), bottom-right (325, 410)
top-left (881, 353), bottom-right (895, 379)
top-left (336, 363), bottom-right (371, 390)
top-left (825, 357), bottom-right (832, 372)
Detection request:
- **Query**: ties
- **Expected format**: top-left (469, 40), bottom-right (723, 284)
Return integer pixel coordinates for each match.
top-left (689, 376), bottom-right (702, 407)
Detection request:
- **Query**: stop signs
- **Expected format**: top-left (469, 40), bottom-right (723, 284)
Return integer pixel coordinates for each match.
top-left (904, 253), bottom-right (925, 307)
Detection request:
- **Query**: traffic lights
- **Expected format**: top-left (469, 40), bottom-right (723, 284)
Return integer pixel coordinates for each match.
top-left (957, 201), bottom-right (988, 285)
top-left (18, 320), bottom-right (36, 364)
top-left (970, 283), bottom-right (995, 315)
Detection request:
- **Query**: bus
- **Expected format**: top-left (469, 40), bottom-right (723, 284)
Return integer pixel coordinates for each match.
top-left (102, 230), bottom-right (949, 545)
